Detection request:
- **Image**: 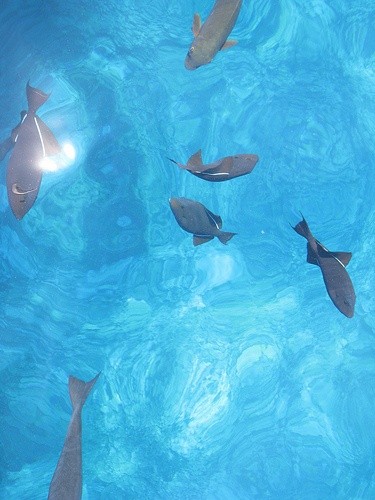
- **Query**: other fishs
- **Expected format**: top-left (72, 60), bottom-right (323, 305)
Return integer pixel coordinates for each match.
top-left (8, 79), bottom-right (51, 222)
top-left (165, 150), bottom-right (259, 182)
top-left (184, 0), bottom-right (242, 70)
top-left (169, 197), bottom-right (237, 247)
top-left (47, 369), bottom-right (101, 500)
top-left (288, 210), bottom-right (356, 319)
